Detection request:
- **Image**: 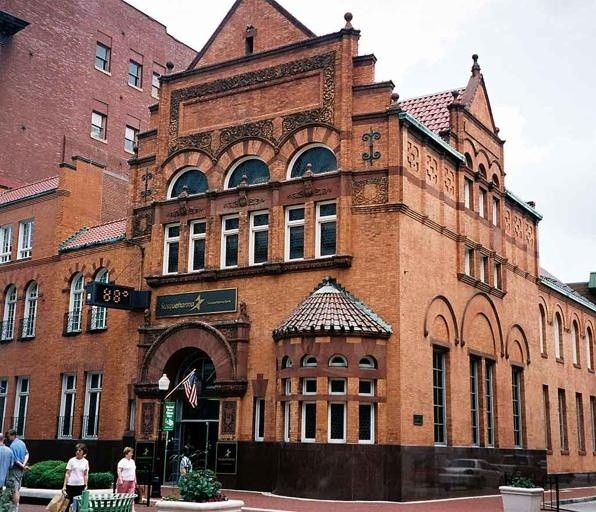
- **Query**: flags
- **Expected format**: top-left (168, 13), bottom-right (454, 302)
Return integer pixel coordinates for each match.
top-left (182, 374), bottom-right (199, 408)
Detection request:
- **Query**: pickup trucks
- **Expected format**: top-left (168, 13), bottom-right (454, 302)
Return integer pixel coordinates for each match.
top-left (495, 454), bottom-right (545, 480)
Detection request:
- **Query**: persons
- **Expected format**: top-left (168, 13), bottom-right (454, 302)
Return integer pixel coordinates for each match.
top-left (0, 433), bottom-right (14, 493)
top-left (178, 446), bottom-right (193, 485)
top-left (83, 454), bottom-right (90, 483)
top-left (6, 429), bottom-right (31, 512)
top-left (61, 443), bottom-right (88, 512)
top-left (116, 446), bottom-right (138, 512)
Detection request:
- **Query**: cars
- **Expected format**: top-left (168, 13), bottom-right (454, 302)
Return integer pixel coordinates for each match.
top-left (440, 457), bottom-right (505, 489)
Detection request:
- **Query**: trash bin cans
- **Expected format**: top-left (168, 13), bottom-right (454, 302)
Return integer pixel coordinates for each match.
top-left (151, 476), bottom-right (162, 498)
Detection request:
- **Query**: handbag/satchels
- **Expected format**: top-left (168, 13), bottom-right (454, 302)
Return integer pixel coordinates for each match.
top-left (45, 495), bottom-right (69, 512)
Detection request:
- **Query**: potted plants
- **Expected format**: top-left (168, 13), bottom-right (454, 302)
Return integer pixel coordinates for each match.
top-left (498, 469), bottom-right (546, 512)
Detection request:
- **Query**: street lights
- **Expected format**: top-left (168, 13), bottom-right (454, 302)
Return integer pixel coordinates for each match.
top-left (150, 373), bottom-right (171, 499)
